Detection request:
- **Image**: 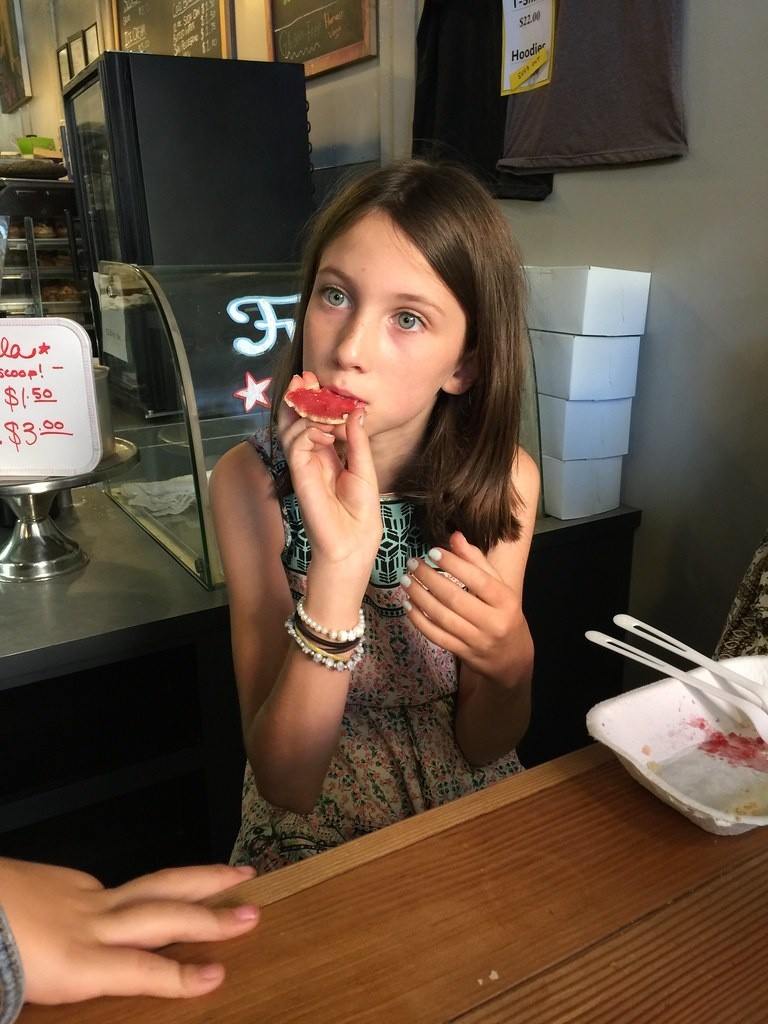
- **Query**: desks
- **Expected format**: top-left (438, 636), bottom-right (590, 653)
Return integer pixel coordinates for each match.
top-left (0, 408), bottom-right (647, 893)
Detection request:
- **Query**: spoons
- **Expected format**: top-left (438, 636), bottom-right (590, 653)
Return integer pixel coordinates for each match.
top-left (585, 631), bottom-right (767, 745)
top-left (613, 613), bottom-right (767, 712)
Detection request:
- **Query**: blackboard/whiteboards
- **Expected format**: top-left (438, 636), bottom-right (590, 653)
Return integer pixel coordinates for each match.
top-left (0, 315), bottom-right (105, 484)
top-left (105, 0), bottom-right (236, 59)
top-left (262, 0), bottom-right (380, 79)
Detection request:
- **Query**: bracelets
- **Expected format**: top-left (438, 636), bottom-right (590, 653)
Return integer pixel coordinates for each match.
top-left (285, 595), bottom-right (366, 671)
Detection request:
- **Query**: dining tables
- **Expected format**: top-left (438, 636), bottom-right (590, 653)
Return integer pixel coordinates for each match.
top-left (2, 737), bottom-right (766, 1024)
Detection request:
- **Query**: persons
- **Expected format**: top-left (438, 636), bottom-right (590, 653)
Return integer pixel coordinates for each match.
top-left (209, 157), bottom-right (540, 876)
top-left (0, 856), bottom-right (258, 1023)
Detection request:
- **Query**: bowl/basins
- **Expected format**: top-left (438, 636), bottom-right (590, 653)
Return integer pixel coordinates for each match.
top-left (585, 653), bottom-right (767, 835)
